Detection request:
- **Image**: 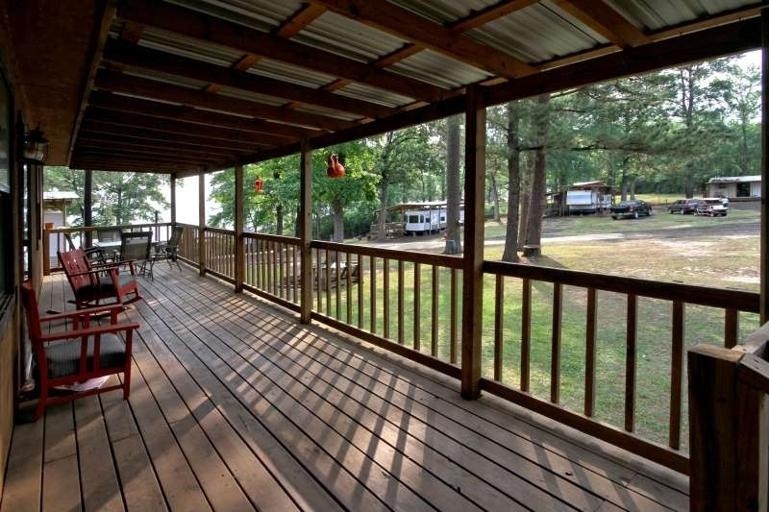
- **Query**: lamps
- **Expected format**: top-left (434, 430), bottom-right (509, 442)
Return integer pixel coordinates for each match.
top-left (255, 162), bottom-right (263, 191)
top-left (327, 145), bottom-right (345, 177)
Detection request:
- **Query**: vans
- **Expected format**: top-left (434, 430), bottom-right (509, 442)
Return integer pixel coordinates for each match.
top-left (667, 198), bottom-right (729, 216)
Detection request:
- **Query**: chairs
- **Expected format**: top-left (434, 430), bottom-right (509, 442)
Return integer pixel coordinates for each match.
top-left (140, 227), bottom-right (183, 278)
top-left (113, 232), bottom-right (153, 281)
top-left (95, 226), bottom-right (138, 272)
top-left (46, 247), bottom-right (143, 319)
top-left (448, 240), bottom-right (459, 254)
top-left (20, 278), bottom-right (140, 423)
top-left (64, 233), bottom-right (108, 277)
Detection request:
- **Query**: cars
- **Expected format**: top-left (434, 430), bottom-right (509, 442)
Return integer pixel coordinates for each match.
top-left (609, 199), bottom-right (652, 218)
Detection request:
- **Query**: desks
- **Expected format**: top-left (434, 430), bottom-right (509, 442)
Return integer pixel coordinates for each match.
top-left (523, 244), bottom-right (541, 257)
top-left (93, 239), bottom-right (159, 281)
